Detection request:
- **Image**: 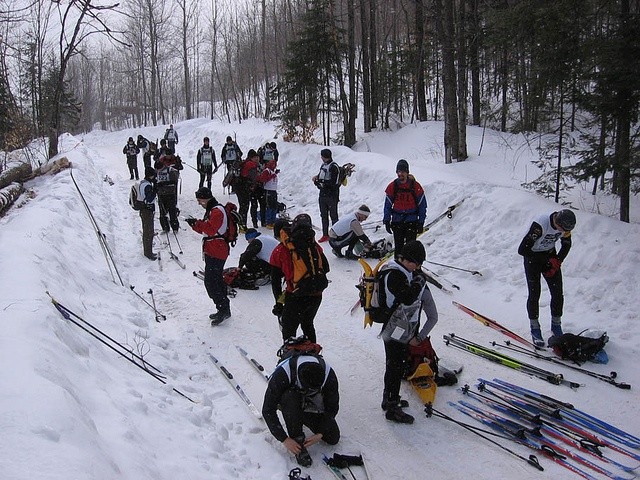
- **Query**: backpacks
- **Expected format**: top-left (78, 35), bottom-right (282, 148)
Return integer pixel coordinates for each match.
top-left (402, 335), bottom-right (439, 385)
top-left (279, 227), bottom-right (328, 297)
top-left (223, 267), bottom-right (240, 285)
top-left (223, 159), bottom-right (250, 193)
top-left (361, 239), bottom-right (392, 259)
top-left (351, 269), bottom-right (408, 339)
top-left (329, 162), bottom-right (356, 187)
top-left (129, 181), bottom-right (151, 210)
top-left (231, 269), bottom-right (259, 290)
top-left (276, 336), bottom-right (326, 398)
top-left (547, 329), bottom-right (609, 366)
top-left (203, 203), bottom-right (243, 247)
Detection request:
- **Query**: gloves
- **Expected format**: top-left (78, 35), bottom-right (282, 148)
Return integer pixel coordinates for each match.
top-left (414, 223), bottom-right (423, 234)
top-left (542, 268), bottom-right (557, 279)
top-left (185, 219), bottom-right (197, 225)
top-left (411, 273), bottom-right (426, 288)
top-left (327, 453), bottom-right (363, 468)
top-left (548, 258), bottom-right (561, 270)
top-left (273, 305), bottom-right (284, 316)
top-left (386, 223), bottom-right (392, 234)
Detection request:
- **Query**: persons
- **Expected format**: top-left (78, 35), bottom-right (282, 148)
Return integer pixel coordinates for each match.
top-left (185, 187), bottom-right (231, 326)
top-left (518, 209), bottom-right (576, 347)
top-left (154, 148), bottom-right (184, 170)
top-left (383, 160), bottom-right (427, 259)
top-left (312, 149), bottom-right (340, 243)
top-left (257, 142), bottom-right (279, 166)
top-left (164, 125), bottom-right (178, 154)
top-left (382, 240), bottom-right (427, 424)
top-left (123, 137), bottom-right (139, 180)
top-left (405, 284), bottom-right (438, 347)
top-left (270, 218), bottom-right (330, 343)
top-left (262, 354), bottom-right (340, 468)
top-left (139, 166), bottom-right (158, 261)
top-left (197, 137), bottom-right (218, 191)
top-left (153, 161), bottom-right (179, 231)
top-left (329, 204), bottom-right (371, 261)
top-left (254, 163), bottom-right (266, 228)
top-left (263, 161), bottom-right (281, 229)
top-left (239, 229), bottom-right (281, 286)
top-left (153, 139), bottom-right (173, 161)
top-left (221, 136), bottom-right (243, 195)
top-left (232, 149), bottom-right (264, 234)
top-left (137, 135), bottom-right (151, 179)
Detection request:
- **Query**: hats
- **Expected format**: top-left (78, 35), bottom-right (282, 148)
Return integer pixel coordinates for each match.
top-left (400, 241), bottom-right (425, 261)
top-left (321, 149), bottom-right (331, 158)
top-left (196, 187), bottom-right (212, 199)
top-left (556, 210), bottom-right (575, 230)
top-left (245, 228), bottom-right (261, 239)
top-left (357, 205), bottom-right (370, 216)
top-left (298, 362), bottom-right (324, 389)
top-left (274, 219), bottom-right (290, 237)
top-left (145, 165), bottom-right (155, 176)
top-left (397, 160), bottom-right (408, 170)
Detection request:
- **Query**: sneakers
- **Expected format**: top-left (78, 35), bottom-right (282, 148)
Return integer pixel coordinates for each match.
top-left (382, 400), bottom-right (409, 410)
top-left (319, 236), bottom-right (329, 242)
top-left (386, 403), bottom-right (414, 423)
top-left (212, 311), bottom-right (231, 325)
top-left (210, 312), bottom-right (219, 319)
top-left (332, 247), bottom-right (344, 257)
top-left (346, 250), bottom-right (361, 260)
top-left (239, 225), bottom-right (248, 233)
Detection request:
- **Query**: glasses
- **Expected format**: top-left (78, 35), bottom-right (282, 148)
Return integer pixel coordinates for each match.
top-left (396, 170), bottom-right (408, 173)
top-left (402, 247), bottom-right (425, 266)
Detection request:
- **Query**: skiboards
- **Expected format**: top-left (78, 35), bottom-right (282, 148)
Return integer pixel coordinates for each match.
top-left (69, 166), bottom-right (123, 285)
top-left (206, 344), bottom-right (270, 420)
top-left (378, 199), bottom-right (462, 261)
top-left (193, 269), bottom-right (238, 299)
top-left (442, 332), bottom-right (580, 392)
top-left (157, 252), bottom-right (186, 272)
top-left (452, 300), bottom-right (547, 352)
top-left (446, 377), bottom-right (640, 480)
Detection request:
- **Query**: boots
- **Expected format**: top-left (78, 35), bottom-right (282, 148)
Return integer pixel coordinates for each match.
top-left (531, 319), bottom-right (544, 347)
top-left (295, 432), bottom-right (312, 468)
top-left (552, 316), bottom-right (563, 336)
